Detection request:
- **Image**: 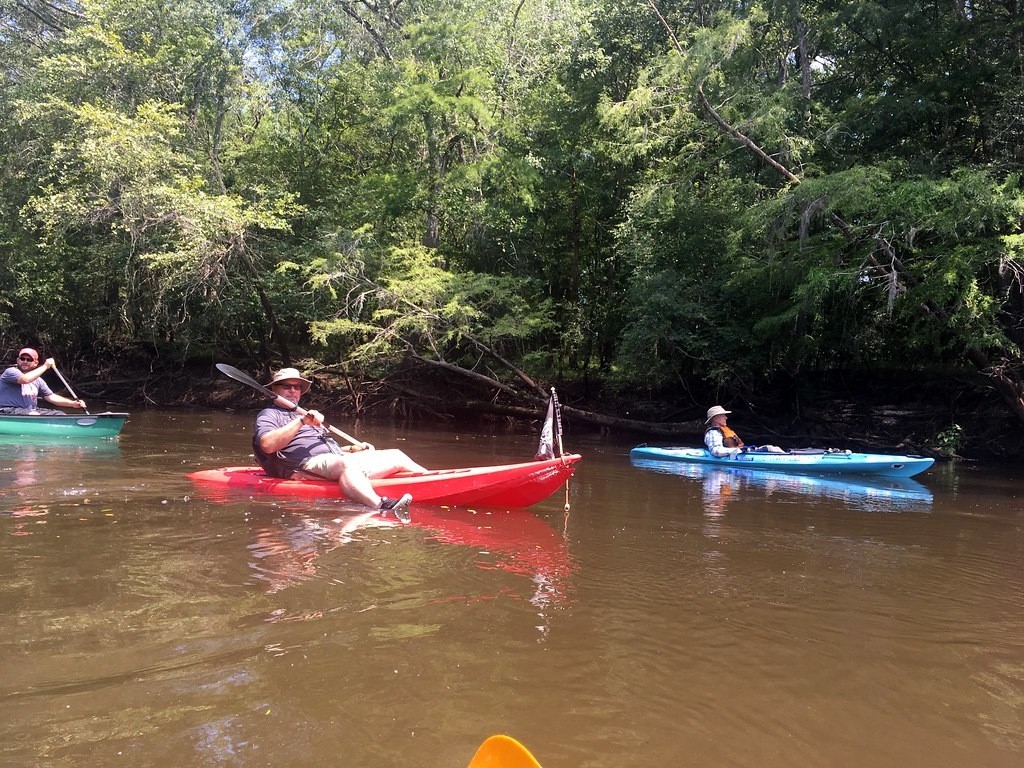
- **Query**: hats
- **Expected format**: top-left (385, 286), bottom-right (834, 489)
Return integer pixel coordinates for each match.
top-left (263, 368), bottom-right (312, 395)
top-left (19, 348), bottom-right (38, 361)
top-left (705, 406), bottom-right (732, 424)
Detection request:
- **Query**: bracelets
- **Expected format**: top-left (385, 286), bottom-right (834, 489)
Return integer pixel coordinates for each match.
top-left (349, 445), bottom-right (354, 454)
top-left (299, 415), bottom-right (305, 425)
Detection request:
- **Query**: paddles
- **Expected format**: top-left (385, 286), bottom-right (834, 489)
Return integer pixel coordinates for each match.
top-left (51, 364), bottom-right (91, 416)
top-left (216, 363), bottom-right (372, 449)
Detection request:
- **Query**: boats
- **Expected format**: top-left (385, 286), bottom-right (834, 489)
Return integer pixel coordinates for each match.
top-left (631, 445), bottom-right (936, 478)
top-left (0, 410), bottom-right (132, 437)
top-left (185, 452), bottom-right (582, 508)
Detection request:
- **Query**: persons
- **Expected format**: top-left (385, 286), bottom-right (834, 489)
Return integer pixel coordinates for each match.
top-left (252, 367), bottom-right (428, 510)
top-left (244, 508), bottom-right (411, 594)
top-left (704, 405), bottom-right (786, 457)
top-left (701, 470), bottom-right (775, 520)
top-left (0, 348), bottom-right (86, 415)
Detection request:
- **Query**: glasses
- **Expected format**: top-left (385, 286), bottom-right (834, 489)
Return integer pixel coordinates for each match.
top-left (275, 382), bottom-right (301, 390)
top-left (20, 357), bottom-right (33, 362)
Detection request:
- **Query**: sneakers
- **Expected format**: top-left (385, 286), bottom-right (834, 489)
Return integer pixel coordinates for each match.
top-left (380, 493), bottom-right (412, 510)
top-left (394, 509), bottom-right (411, 524)
